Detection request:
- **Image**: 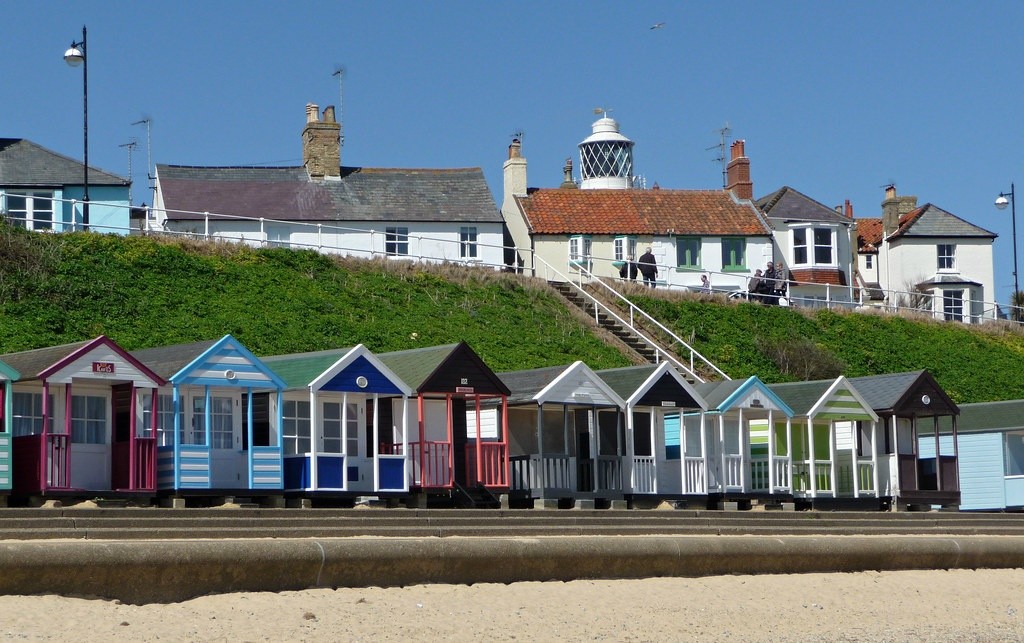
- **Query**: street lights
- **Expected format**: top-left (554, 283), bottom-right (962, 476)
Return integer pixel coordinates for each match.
top-left (63, 24), bottom-right (91, 232)
top-left (994, 182), bottom-right (1019, 323)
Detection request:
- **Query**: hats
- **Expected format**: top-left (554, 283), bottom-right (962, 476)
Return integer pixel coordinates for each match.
top-left (756, 269), bottom-right (762, 273)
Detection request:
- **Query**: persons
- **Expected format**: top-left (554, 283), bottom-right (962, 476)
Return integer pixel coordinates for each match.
top-left (748, 262), bottom-right (786, 305)
top-left (637, 247), bottom-right (658, 288)
top-left (699, 275), bottom-right (710, 294)
top-left (619, 255), bottom-right (638, 282)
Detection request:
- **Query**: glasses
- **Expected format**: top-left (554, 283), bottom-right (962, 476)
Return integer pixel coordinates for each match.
top-left (767, 265), bottom-right (772, 267)
